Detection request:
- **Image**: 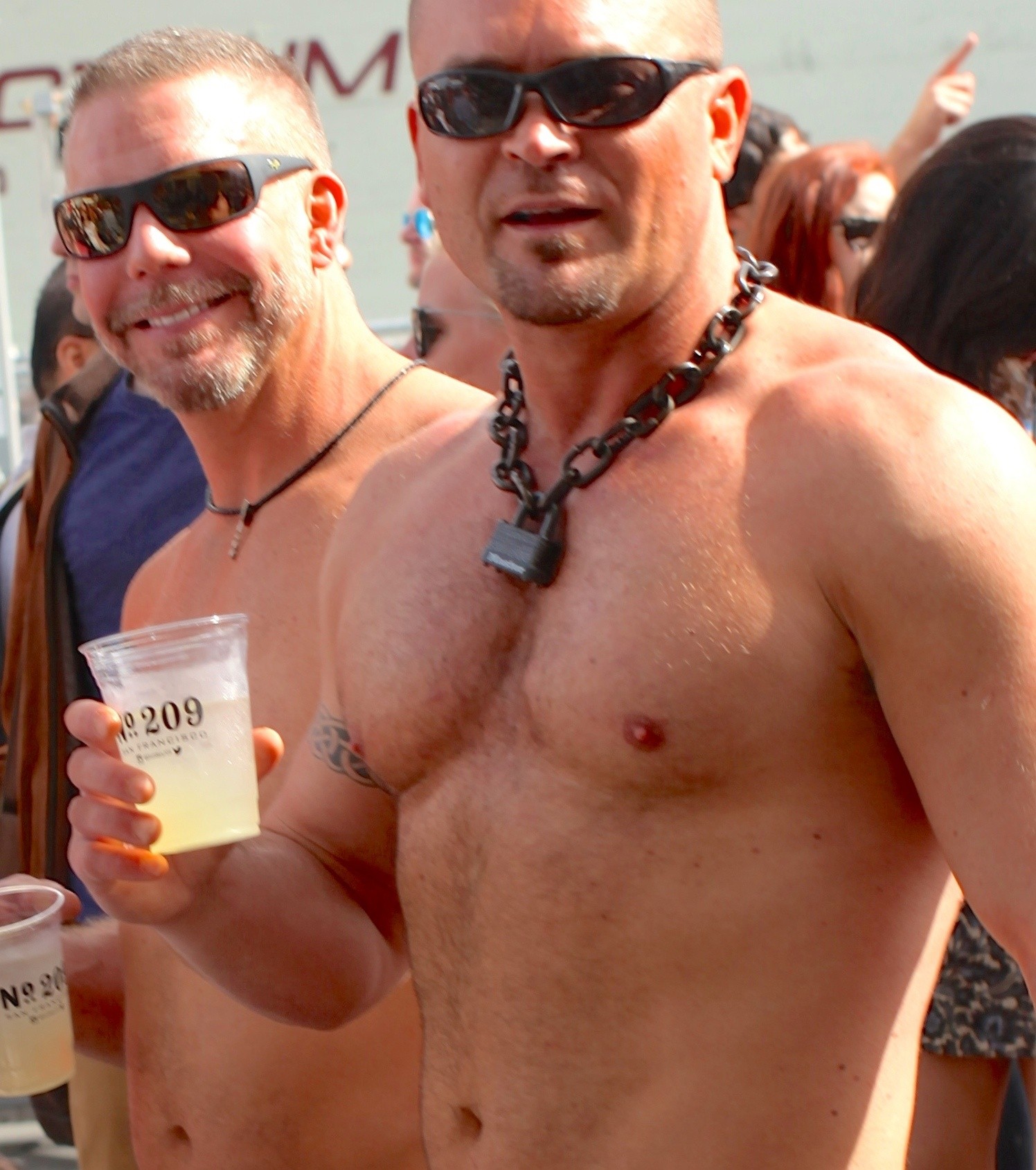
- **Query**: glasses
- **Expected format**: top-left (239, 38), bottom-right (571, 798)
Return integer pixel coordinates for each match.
top-left (399, 210), bottom-right (438, 240)
top-left (418, 54), bottom-right (720, 146)
top-left (51, 152), bottom-right (315, 261)
top-left (829, 217), bottom-right (879, 242)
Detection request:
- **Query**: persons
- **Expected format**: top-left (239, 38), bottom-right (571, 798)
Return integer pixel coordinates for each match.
top-left (751, 139), bottom-right (902, 323)
top-left (400, 189), bottom-right (439, 290)
top-left (840, 113), bottom-right (1035, 1170)
top-left (66, 3), bottom-right (1036, 1169)
top-left (723, 31), bottom-right (978, 265)
top-left (397, 243), bottom-right (508, 400)
top-left (0, 28), bottom-right (502, 1170)
top-left (1, 260), bottom-right (104, 777)
top-left (1, 223), bottom-right (214, 1169)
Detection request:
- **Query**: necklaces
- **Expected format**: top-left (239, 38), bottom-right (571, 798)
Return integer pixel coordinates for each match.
top-left (481, 246), bottom-right (779, 587)
top-left (202, 359), bottom-right (428, 557)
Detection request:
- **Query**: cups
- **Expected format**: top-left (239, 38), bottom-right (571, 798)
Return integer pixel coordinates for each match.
top-left (1, 885), bottom-right (82, 1100)
top-left (79, 612), bottom-right (266, 857)
